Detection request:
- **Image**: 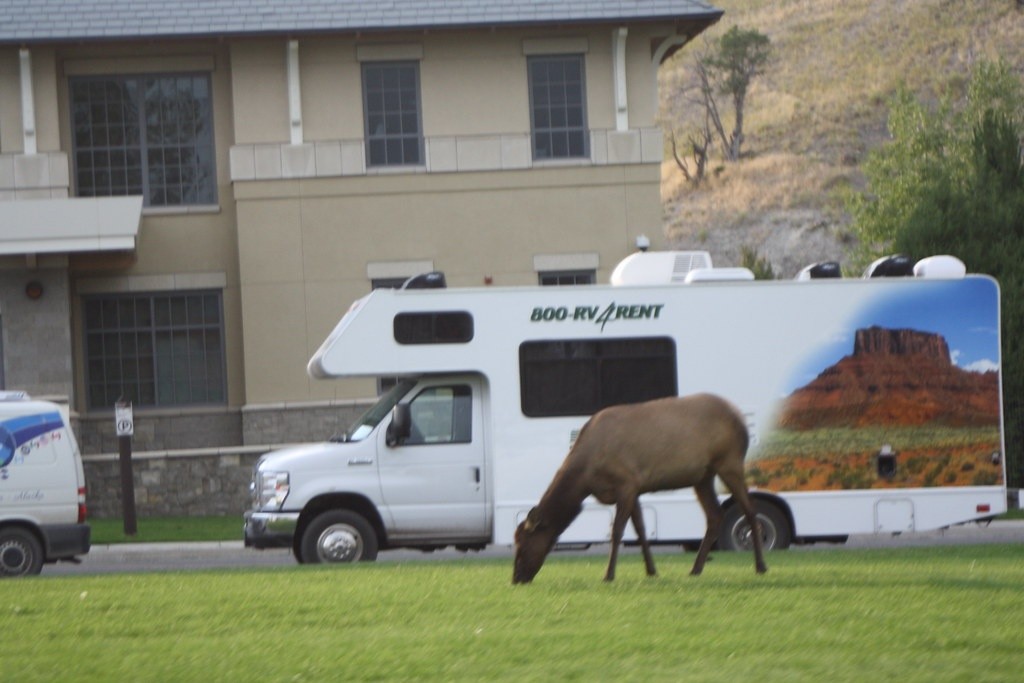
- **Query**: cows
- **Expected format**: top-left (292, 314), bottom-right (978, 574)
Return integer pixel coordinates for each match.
top-left (511, 391), bottom-right (769, 585)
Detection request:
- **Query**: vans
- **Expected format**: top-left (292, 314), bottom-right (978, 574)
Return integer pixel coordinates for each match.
top-left (0, 390), bottom-right (91, 577)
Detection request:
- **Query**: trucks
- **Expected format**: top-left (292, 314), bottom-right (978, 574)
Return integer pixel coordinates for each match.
top-left (243, 251), bottom-right (1008, 564)
